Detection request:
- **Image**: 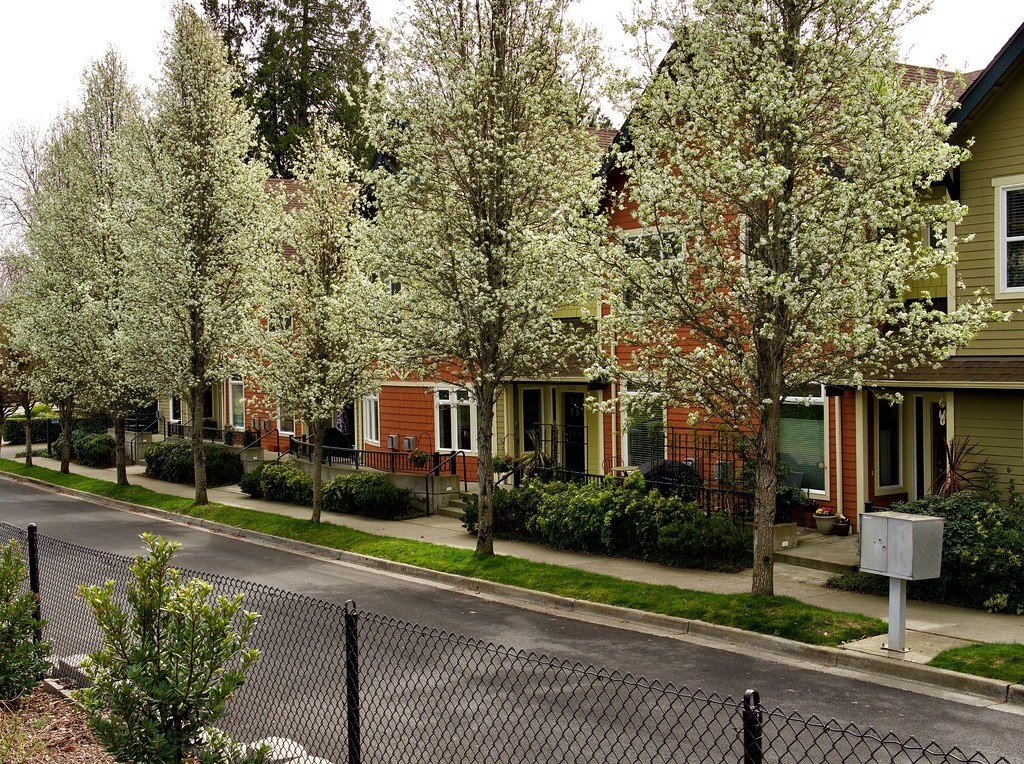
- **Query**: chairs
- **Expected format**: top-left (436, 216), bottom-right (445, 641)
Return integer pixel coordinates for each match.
top-left (602, 457), bottom-right (624, 478)
top-left (786, 472), bottom-right (806, 489)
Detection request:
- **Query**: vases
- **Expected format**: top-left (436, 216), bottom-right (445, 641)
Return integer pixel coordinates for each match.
top-left (494, 466), bottom-right (508, 472)
top-left (414, 461), bottom-right (424, 467)
top-left (834, 523), bottom-right (850, 536)
top-left (813, 513), bottom-right (837, 533)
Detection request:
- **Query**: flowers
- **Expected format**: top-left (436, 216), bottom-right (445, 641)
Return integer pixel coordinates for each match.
top-left (407, 448), bottom-right (428, 462)
top-left (811, 499), bottom-right (833, 515)
top-left (836, 518), bottom-right (850, 523)
top-left (491, 452), bottom-right (514, 472)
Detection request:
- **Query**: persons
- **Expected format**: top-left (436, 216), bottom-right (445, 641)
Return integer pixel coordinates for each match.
top-left (336, 403), bottom-right (354, 448)
top-left (462, 429), bottom-right (470, 449)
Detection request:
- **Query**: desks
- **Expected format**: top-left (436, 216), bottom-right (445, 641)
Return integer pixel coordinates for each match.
top-left (610, 466), bottom-right (638, 476)
